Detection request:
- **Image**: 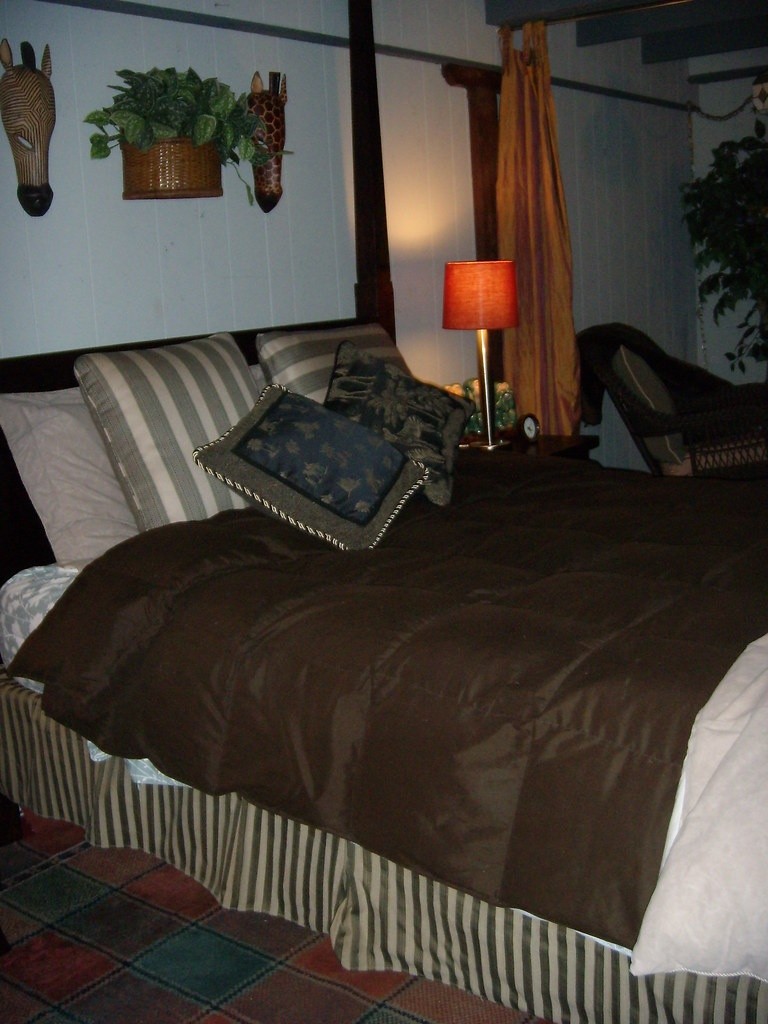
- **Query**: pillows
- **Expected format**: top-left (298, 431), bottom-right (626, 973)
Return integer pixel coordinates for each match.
top-left (192, 383), bottom-right (429, 550)
top-left (1, 363), bottom-right (286, 571)
top-left (74, 332), bottom-right (258, 533)
top-left (256, 323), bottom-right (413, 405)
top-left (324, 340), bottom-right (475, 505)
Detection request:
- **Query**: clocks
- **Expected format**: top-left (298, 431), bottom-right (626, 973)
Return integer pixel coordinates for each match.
top-left (517, 413), bottom-right (540, 442)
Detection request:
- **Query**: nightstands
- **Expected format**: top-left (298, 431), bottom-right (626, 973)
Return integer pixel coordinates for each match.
top-left (458, 435), bottom-right (600, 459)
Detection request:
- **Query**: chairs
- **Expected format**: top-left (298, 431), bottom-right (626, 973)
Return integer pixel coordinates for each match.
top-left (586, 323), bottom-right (768, 478)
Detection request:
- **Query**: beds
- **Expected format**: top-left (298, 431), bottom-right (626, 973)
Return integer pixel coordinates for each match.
top-left (0, 317), bottom-right (768, 1024)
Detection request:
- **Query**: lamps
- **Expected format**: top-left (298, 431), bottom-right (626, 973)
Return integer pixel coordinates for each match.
top-left (443, 261), bottom-right (519, 451)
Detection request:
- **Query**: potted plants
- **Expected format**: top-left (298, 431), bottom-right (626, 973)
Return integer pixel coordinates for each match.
top-left (683, 118), bottom-right (768, 372)
top-left (84, 66), bottom-right (293, 204)
